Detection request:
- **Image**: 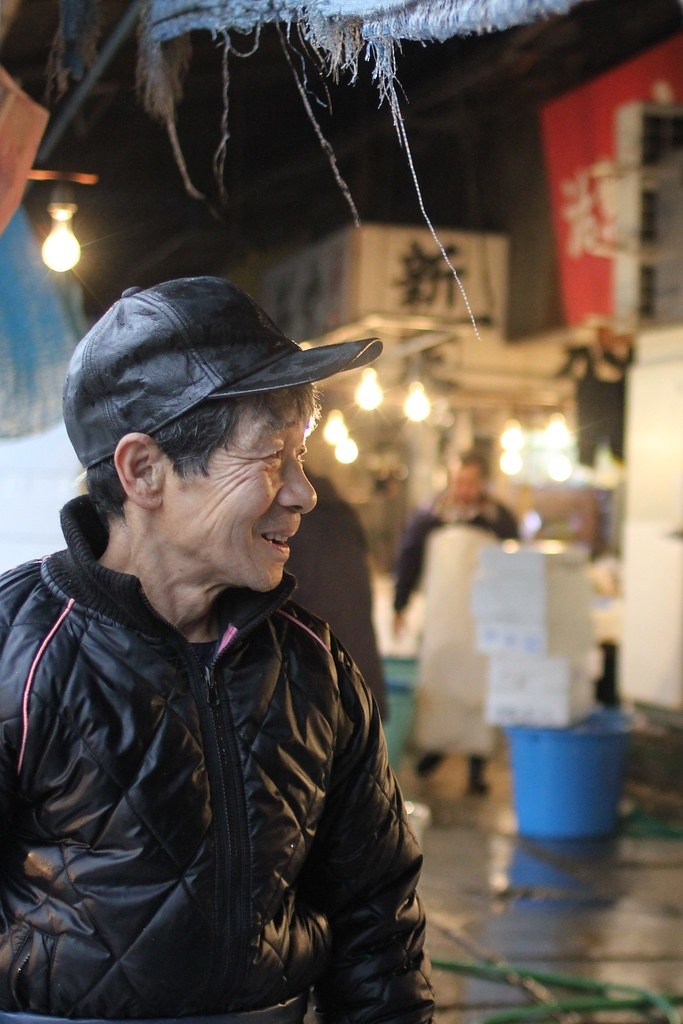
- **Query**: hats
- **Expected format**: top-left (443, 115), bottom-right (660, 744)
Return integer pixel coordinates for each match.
top-left (62, 276), bottom-right (383, 470)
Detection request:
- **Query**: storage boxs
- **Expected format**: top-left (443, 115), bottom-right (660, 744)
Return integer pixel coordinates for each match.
top-left (472, 550), bottom-right (596, 726)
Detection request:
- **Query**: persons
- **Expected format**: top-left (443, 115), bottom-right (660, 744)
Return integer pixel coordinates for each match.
top-left (282, 455), bottom-right (432, 850)
top-left (0, 276), bottom-right (438, 1023)
top-left (550, 317), bottom-right (638, 470)
top-left (392, 453), bottom-right (522, 796)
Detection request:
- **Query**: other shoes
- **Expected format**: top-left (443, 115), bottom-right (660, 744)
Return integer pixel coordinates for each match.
top-left (415, 752), bottom-right (442, 776)
top-left (470, 768), bottom-right (487, 794)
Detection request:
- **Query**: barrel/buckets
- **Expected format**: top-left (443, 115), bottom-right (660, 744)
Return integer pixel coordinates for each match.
top-left (503, 708), bottom-right (633, 841)
